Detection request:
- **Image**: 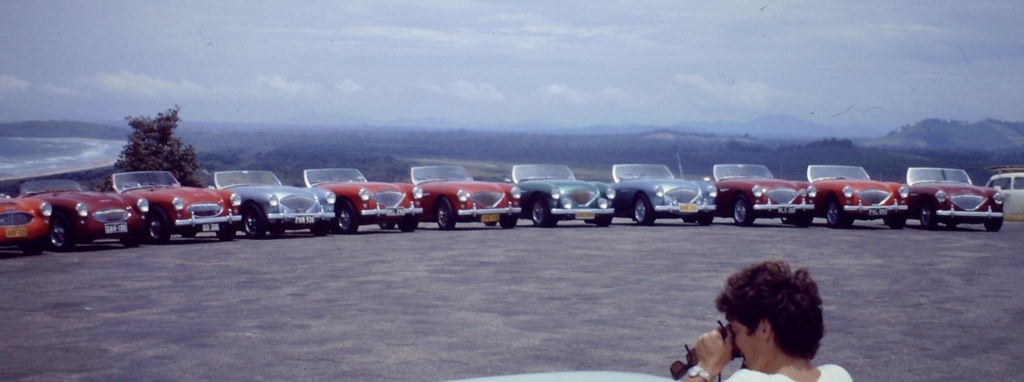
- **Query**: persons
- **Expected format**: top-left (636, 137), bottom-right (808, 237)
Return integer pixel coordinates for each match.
top-left (683, 260), bottom-right (854, 382)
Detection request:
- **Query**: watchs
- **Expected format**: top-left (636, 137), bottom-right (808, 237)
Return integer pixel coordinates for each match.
top-left (687, 366), bottom-right (712, 382)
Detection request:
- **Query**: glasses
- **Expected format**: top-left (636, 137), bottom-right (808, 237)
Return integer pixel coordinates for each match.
top-left (670, 345), bottom-right (698, 380)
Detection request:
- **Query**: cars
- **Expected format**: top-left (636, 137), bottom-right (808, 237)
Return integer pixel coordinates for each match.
top-left (704, 164), bottom-right (818, 228)
top-left (0, 191), bottom-right (53, 255)
top-left (607, 163), bottom-right (718, 226)
top-left (302, 168), bottom-right (424, 234)
top-left (806, 164), bottom-right (910, 230)
top-left (504, 163), bottom-right (616, 228)
top-left (903, 166), bottom-right (1006, 232)
top-left (97, 170), bottom-right (244, 246)
top-left (985, 164), bottom-right (1024, 214)
top-left (15, 178), bottom-right (150, 253)
top-left (409, 166), bottom-right (523, 231)
top-left (207, 169), bottom-right (337, 240)
